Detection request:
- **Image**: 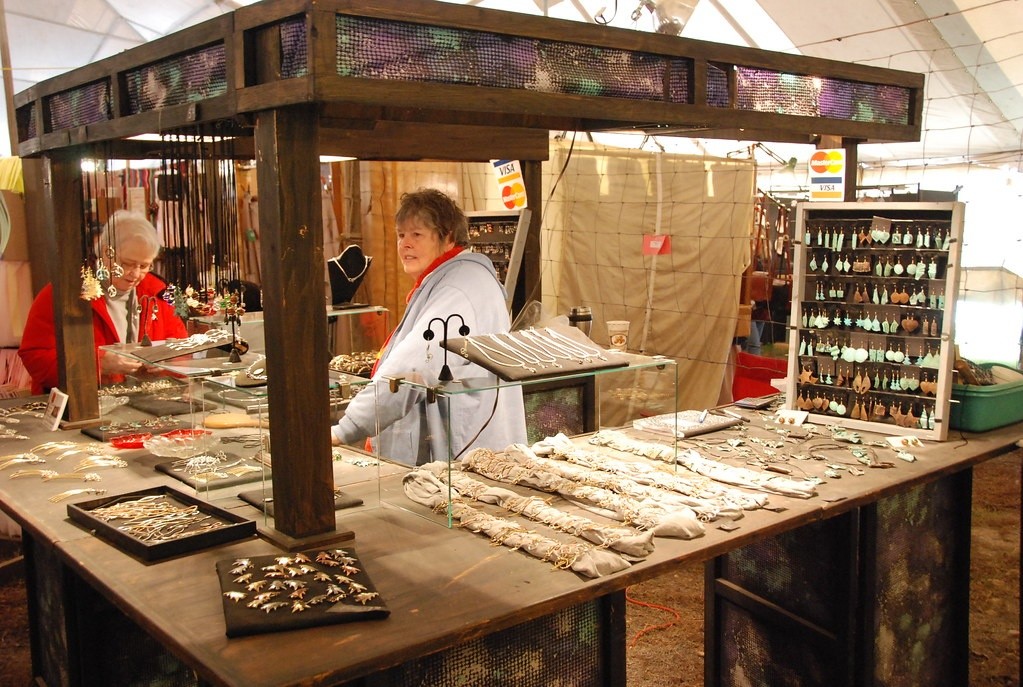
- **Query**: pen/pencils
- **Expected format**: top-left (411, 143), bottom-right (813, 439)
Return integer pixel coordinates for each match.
top-left (699, 409), bottom-right (708, 423)
top-left (720, 408), bottom-right (751, 423)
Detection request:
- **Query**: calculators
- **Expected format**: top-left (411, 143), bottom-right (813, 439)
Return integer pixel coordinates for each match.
top-left (734, 397), bottom-right (778, 409)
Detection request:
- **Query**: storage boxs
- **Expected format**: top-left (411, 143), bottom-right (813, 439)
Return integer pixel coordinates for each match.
top-left (950, 362), bottom-right (1023, 432)
top-left (464, 208), bottom-right (532, 312)
top-left (786, 201), bottom-right (965, 441)
top-left (66, 485), bottom-right (257, 563)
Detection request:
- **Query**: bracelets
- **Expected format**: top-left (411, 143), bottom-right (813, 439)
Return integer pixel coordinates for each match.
top-left (329, 355), bottom-right (374, 375)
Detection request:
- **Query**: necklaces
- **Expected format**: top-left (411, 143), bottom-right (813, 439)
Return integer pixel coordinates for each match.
top-left (334, 256), bottom-right (368, 282)
top-left (161, 120), bottom-right (246, 343)
top-left (466, 328), bottom-right (608, 375)
top-left (87, 497), bottom-right (232, 543)
top-left (219, 358), bottom-right (275, 412)
top-left (87, 140), bottom-right (124, 299)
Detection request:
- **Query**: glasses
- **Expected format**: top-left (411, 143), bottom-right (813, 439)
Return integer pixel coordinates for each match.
top-left (116, 254), bottom-right (154, 273)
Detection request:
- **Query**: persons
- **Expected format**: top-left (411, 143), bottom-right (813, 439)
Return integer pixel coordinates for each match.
top-left (17, 210), bottom-right (192, 396)
top-left (331, 188), bottom-right (529, 467)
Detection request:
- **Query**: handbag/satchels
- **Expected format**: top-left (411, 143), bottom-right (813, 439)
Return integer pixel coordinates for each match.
top-left (733, 198), bottom-right (792, 339)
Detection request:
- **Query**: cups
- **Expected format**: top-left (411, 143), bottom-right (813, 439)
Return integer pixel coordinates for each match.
top-left (606, 320), bottom-right (630, 353)
top-left (568, 305), bottom-right (593, 337)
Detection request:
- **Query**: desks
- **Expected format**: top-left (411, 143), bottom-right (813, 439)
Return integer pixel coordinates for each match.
top-left (0, 380), bottom-right (1022, 686)
